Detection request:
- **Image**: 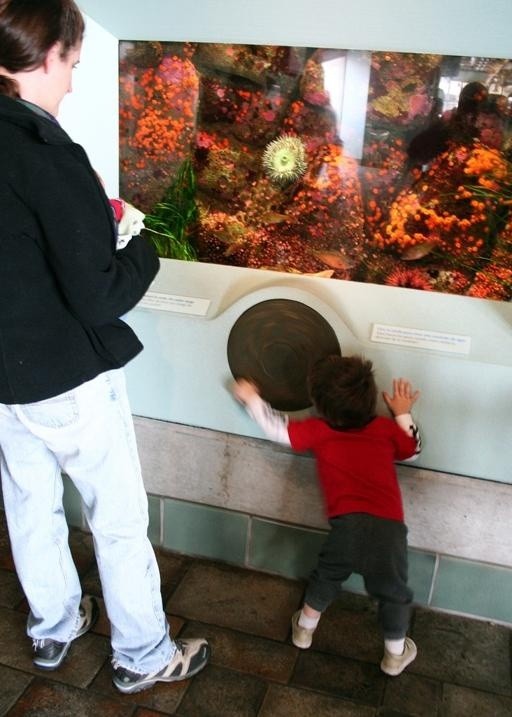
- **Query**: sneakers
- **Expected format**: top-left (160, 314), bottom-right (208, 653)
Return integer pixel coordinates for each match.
top-left (380, 637), bottom-right (418, 676)
top-left (112, 637), bottom-right (211, 694)
top-left (291, 609), bottom-right (317, 649)
top-left (31, 594), bottom-right (101, 671)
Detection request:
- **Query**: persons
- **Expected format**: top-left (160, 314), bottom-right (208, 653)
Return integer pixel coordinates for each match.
top-left (0, 0), bottom-right (212, 695)
top-left (227, 355), bottom-right (422, 677)
